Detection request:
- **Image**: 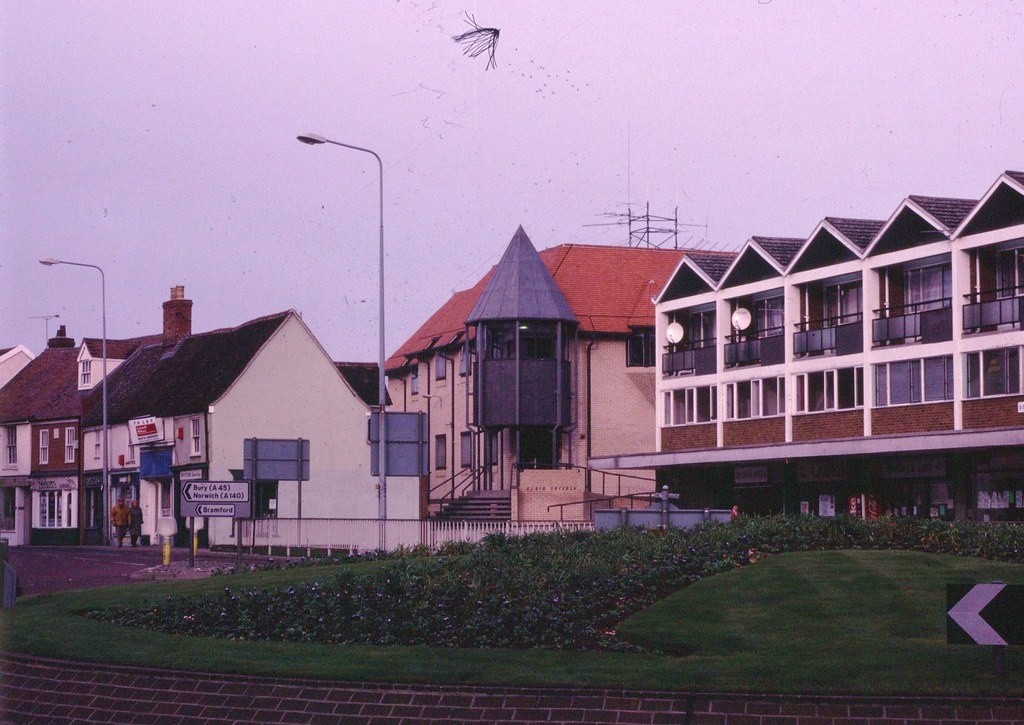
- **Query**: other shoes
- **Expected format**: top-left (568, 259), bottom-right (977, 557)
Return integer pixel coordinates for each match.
top-left (132, 544), bottom-right (139, 547)
top-left (119, 542), bottom-right (122, 547)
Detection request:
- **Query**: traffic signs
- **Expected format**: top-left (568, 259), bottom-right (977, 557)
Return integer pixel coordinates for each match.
top-left (180, 481), bottom-right (251, 520)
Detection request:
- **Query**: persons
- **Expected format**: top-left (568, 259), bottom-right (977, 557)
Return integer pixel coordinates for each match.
top-left (729, 504), bottom-right (741, 520)
top-left (128, 500), bottom-right (144, 547)
top-left (110, 498), bottom-right (132, 547)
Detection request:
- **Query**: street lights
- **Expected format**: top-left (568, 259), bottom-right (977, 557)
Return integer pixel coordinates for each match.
top-left (38, 257), bottom-right (110, 547)
top-left (295, 132), bottom-right (387, 520)
